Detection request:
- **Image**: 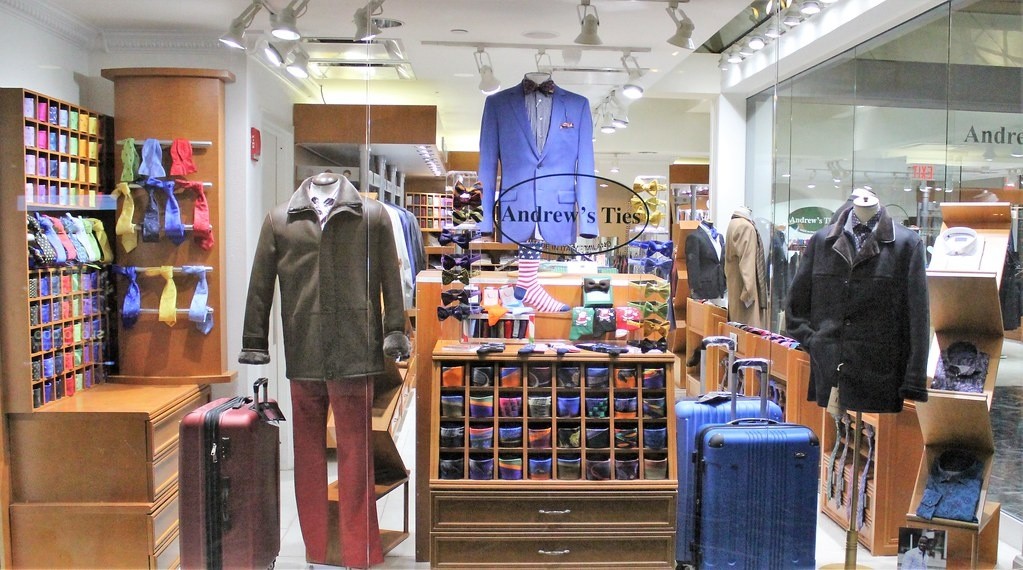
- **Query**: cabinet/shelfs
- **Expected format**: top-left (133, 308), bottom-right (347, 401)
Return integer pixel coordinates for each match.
top-left (715, 319), bottom-right (822, 448)
top-left (0, 85), bottom-right (109, 413)
top-left (405, 191), bottom-right (456, 270)
top-left (901, 201), bottom-right (1015, 570)
top-left (685, 298), bottom-right (728, 400)
top-left (430, 337), bottom-right (681, 569)
top-left (10, 382), bottom-right (213, 570)
top-left (818, 394), bottom-right (925, 555)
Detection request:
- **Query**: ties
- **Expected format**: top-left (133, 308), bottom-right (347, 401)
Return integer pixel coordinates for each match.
top-left (26, 137), bottom-right (214, 334)
top-left (826, 413), bottom-right (875, 531)
top-left (851, 211), bottom-right (881, 252)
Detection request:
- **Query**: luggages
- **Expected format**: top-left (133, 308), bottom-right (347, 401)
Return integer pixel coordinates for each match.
top-left (675, 336), bottom-right (819, 570)
top-left (177, 377), bottom-right (284, 568)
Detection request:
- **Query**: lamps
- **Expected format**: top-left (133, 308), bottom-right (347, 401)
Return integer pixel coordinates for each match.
top-left (217, 0), bottom-right (841, 145)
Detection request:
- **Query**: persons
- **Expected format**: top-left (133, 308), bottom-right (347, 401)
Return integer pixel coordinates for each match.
top-left (238, 173), bottom-right (409, 570)
top-left (725, 206), bottom-right (767, 328)
top-left (476, 72), bottom-right (599, 245)
top-left (686, 220), bottom-right (728, 299)
top-left (786, 195), bottom-right (931, 413)
top-left (901, 535), bottom-right (930, 570)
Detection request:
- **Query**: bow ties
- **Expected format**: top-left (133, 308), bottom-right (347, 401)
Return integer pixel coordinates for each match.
top-left (628, 177), bottom-right (668, 354)
top-left (436, 180), bottom-right (484, 323)
top-left (523, 78), bottom-right (554, 97)
top-left (711, 230), bottom-right (718, 240)
top-left (584, 278), bottom-right (610, 294)
